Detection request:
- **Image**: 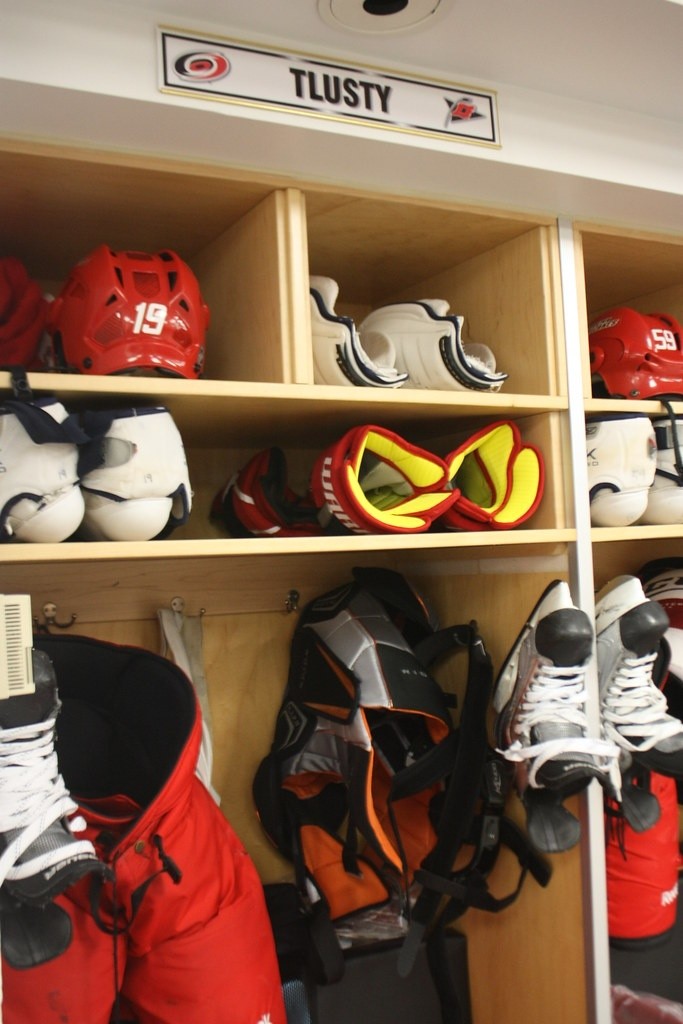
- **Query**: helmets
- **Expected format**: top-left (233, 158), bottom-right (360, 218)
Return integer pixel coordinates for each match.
top-left (589, 306), bottom-right (683, 401)
top-left (43, 242), bottom-right (212, 379)
top-left (0, 242), bottom-right (48, 380)
top-left (309, 270), bottom-right (408, 394)
top-left (640, 445), bottom-right (683, 524)
top-left (585, 415), bottom-right (658, 526)
top-left (359, 298), bottom-right (510, 393)
top-left (76, 405), bottom-right (194, 542)
top-left (0, 392), bottom-right (86, 543)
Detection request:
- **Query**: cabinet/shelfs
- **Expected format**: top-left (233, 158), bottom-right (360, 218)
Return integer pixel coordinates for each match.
top-left (0, 133), bottom-right (683, 1024)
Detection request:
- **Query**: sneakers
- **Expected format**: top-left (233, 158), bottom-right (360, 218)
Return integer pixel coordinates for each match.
top-left (0, 649), bottom-right (116, 971)
top-left (490, 579), bottom-right (621, 854)
top-left (594, 573), bottom-right (682, 832)
